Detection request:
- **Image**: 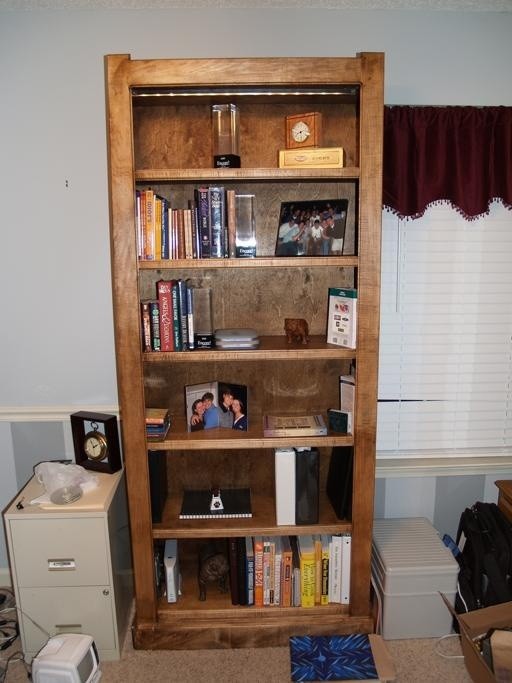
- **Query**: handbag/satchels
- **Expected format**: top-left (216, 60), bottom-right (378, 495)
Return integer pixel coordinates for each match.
top-left (454, 501), bottom-right (512, 614)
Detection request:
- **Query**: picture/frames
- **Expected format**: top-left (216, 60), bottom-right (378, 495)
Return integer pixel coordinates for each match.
top-left (185, 379), bottom-right (249, 434)
top-left (273, 197), bottom-right (349, 255)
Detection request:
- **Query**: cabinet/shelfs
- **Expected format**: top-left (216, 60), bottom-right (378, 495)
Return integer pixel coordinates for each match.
top-left (103, 51), bottom-right (385, 654)
top-left (4, 468), bottom-right (136, 664)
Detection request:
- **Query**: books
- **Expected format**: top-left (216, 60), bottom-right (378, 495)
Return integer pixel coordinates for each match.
top-left (145, 408), bottom-right (172, 442)
top-left (262, 414), bottom-right (328, 438)
top-left (178, 488), bottom-right (252, 520)
top-left (135, 185), bottom-right (236, 261)
top-left (226, 536), bottom-right (351, 609)
top-left (140, 278), bottom-right (211, 353)
top-left (327, 374), bottom-right (355, 437)
top-left (326, 287), bottom-right (358, 349)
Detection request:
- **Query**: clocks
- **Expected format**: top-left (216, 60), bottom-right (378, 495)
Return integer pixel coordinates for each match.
top-left (83, 430), bottom-right (107, 462)
top-left (285, 110), bottom-right (322, 149)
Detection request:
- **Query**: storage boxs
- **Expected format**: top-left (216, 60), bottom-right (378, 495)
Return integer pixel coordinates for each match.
top-left (372, 515), bottom-right (511, 682)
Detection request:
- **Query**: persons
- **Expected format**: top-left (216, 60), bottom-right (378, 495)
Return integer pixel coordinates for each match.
top-left (217, 390), bottom-right (247, 433)
top-left (275, 201), bottom-right (345, 255)
top-left (190, 393), bottom-right (218, 433)
